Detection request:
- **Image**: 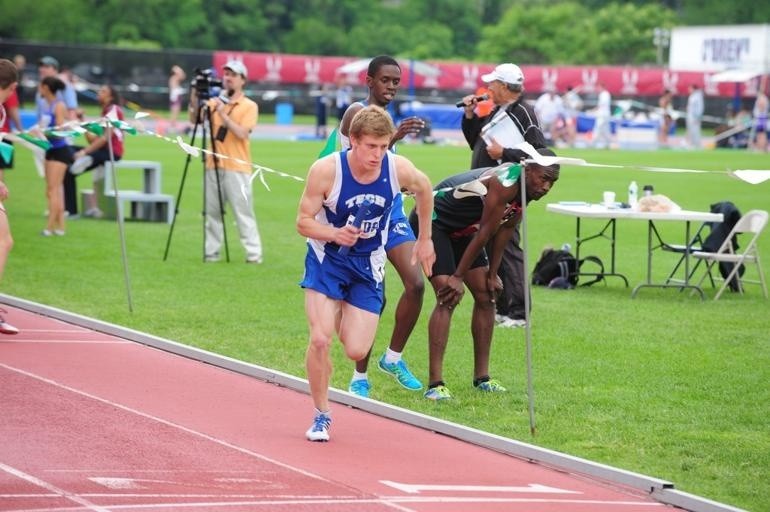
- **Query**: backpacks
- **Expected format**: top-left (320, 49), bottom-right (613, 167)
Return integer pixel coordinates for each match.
top-left (531, 248), bottom-right (604, 289)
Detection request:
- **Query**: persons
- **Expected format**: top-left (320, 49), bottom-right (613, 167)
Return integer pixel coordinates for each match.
top-left (315, 84), bottom-right (329, 138)
top-left (685, 83), bottom-right (706, 148)
top-left (36, 56), bottom-right (79, 220)
top-left (408, 148), bottom-right (560, 402)
top-left (2, 54), bottom-right (26, 132)
top-left (295, 103), bottom-right (437, 441)
top-left (456, 63), bottom-right (547, 329)
top-left (592, 79), bottom-right (613, 148)
top-left (339, 55), bottom-right (425, 398)
top-left (534, 83), bottom-right (568, 146)
top-left (190, 60), bottom-right (263, 264)
top-left (39, 76), bottom-right (70, 237)
top-left (200, 66), bottom-right (221, 97)
top-left (336, 73), bottom-right (353, 120)
top-left (64, 83), bottom-right (124, 219)
top-left (659, 89), bottom-right (673, 147)
top-left (562, 84), bottom-right (584, 144)
top-left (1, 59), bottom-right (20, 334)
top-left (168, 64), bottom-right (189, 127)
top-left (714, 90), bottom-right (770, 150)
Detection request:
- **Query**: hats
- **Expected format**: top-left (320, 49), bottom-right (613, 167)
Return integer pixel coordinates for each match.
top-left (481, 64), bottom-right (524, 84)
top-left (38, 55), bottom-right (58, 65)
top-left (223, 61), bottom-right (247, 79)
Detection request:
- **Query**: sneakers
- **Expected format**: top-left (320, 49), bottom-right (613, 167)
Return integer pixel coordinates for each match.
top-left (473, 379), bottom-right (505, 393)
top-left (305, 408), bottom-right (332, 441)
top-left (204, 250), bottom-right (219, 263)
top-left (349, 377), bottom-right (370, 398)
top-left (424, 386), bottom-right (451, 400)
top-left (498, 317), bottom-right (527, 328)
top-left (377, 353), bottom-right (423, 390)
top-left (494, 314), bottom-right (508, 322)
top-left (245, 253), bottom-right (263, 263)
top-left (0, 316), bottom-right (20, 335)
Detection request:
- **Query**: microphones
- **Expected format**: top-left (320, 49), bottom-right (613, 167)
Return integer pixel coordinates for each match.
top-left (456, 93), bottom-right (490, 108)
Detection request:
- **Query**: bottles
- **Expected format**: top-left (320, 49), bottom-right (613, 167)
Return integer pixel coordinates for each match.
top-left (643, 185), bottom-right (654, 197)
top-left (628, 181), bottom-right (638, 207)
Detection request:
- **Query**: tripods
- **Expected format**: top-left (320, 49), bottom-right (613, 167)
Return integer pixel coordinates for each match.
top-left (163, 93), bottom-right (230, 263)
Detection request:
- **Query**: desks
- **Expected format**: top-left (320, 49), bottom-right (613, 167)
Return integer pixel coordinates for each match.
top-left (546, 204), bottom-right (724, 300)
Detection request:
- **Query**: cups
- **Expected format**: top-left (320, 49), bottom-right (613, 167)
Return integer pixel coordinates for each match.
top-left (603, 191), bottom-right (616, 204)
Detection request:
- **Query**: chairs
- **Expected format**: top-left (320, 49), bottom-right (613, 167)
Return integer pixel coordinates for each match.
top-left (690, 210), bottom-right (768, 300)
top-left (662, 203), bottom-right (736, 291)
